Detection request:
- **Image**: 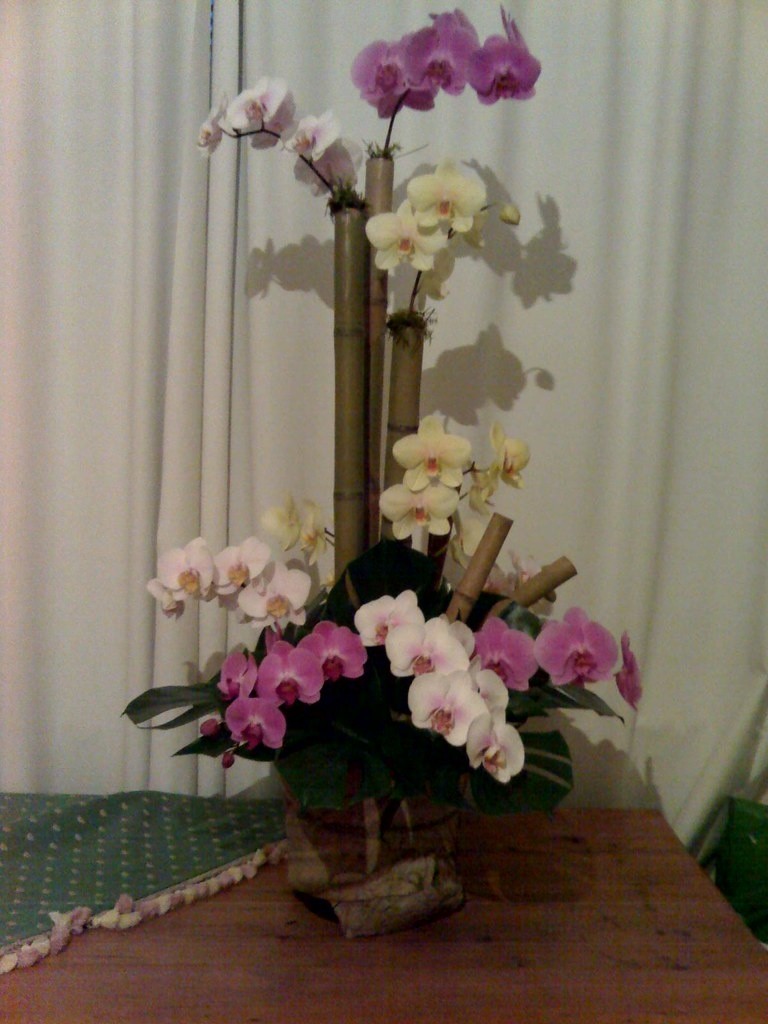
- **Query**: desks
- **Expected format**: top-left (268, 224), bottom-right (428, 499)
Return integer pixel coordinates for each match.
top-left (0, 792), bottom-right (768, 1024)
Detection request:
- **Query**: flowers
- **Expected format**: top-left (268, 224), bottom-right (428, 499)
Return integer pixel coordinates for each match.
top-left (122, 5), bottom-right (640, 818)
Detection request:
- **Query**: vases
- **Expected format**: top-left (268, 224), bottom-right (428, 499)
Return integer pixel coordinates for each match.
top-left (276, 770), bottom-right (465, 937)
